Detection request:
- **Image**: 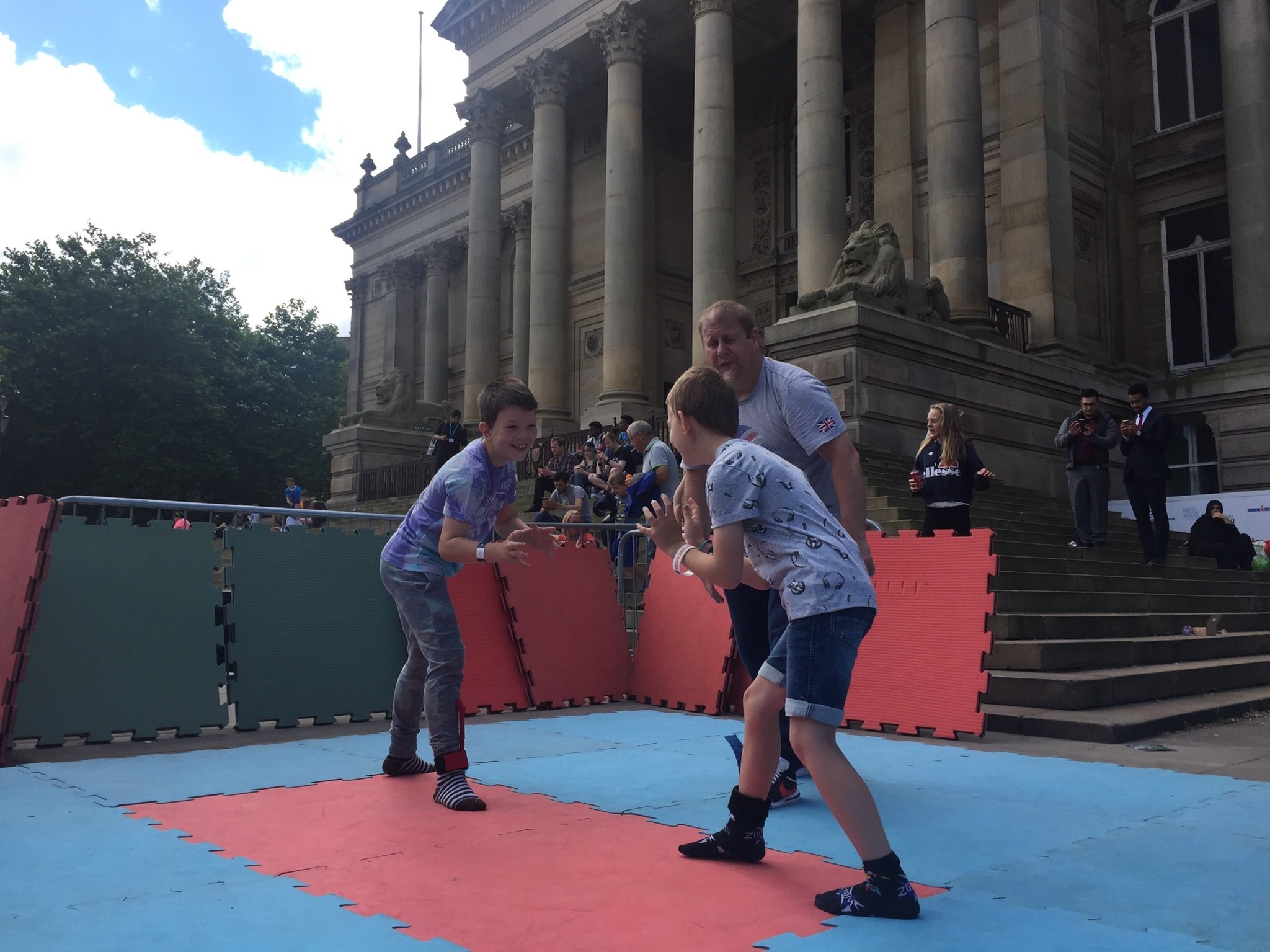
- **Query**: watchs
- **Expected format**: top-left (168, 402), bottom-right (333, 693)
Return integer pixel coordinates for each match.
top-left (604, 458), bottom-right (611, 465)
top-left (1136, 429), bottom-right (1141, 436)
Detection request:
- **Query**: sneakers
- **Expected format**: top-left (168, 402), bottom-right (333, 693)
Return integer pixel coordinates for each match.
top-left (1134, 556), bottom-right (1167, 567)
top-left (770, 773), bottom-right (801, 809)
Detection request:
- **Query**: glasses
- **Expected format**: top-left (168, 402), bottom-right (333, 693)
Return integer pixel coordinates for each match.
top-left (1080, 401), bottom-right (1098, 407)
top-left (583, 448), bottom-right (592, 453)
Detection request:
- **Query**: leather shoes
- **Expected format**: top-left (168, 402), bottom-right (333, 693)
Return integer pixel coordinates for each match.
top-left (524, 503), bottom-right (542, 513)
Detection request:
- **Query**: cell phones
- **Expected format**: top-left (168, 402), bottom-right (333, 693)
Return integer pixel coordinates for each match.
top-left (542, 497), bottom-right (547, 500)
top-left (537, 464), bottom-right (544, 469)
top-left (1078, 419), bottom-right (1089, 431)
top-left (1123, 420), bottom-right (1130, 425)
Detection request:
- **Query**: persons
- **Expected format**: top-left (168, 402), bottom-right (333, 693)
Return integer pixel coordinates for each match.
top-left (1055, 390), bottom-right (1116, 549)
top-left (1190, 501), bottom-right (1256, 569)
top-left (908, 402), bottom-right (997, 539)
top-left (1118, 383), bottom-right (1173, 568)
top-left (173, 378), bottom-right (682, 811)
top-left (680, 301), bottom-right (874, 810)
top-left (636, 368), bottom-right (920, 921)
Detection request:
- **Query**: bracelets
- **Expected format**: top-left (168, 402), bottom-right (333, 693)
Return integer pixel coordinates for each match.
top-left (671, 538), bottom-right (714, 577)
top-left (476, 542), bottom-right (488, 563)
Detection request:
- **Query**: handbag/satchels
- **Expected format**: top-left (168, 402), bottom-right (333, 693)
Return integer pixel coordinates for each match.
top-left (426, 420), bottom-right (447, 455)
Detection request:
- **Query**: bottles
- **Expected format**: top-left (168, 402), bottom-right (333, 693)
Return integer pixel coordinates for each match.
top-left (353, 508), bottom-right (362, 511)
top-left (1251, 538), bottom-right (1265, 547)
top-left (623, 471), bottom-right (633, 480)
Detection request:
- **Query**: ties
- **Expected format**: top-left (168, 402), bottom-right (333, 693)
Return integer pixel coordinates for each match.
top-left (1137, 414), bottom-right (1144, 430)
